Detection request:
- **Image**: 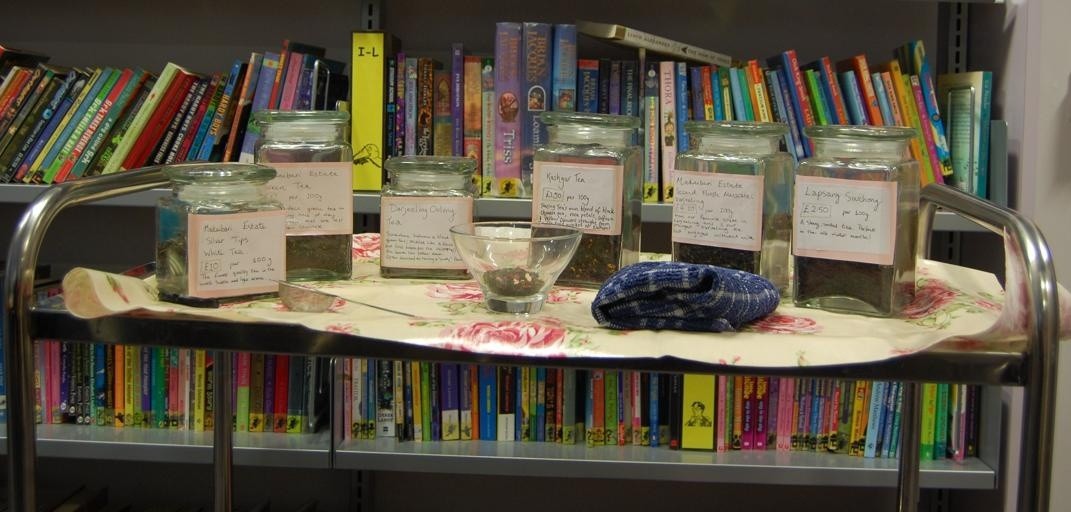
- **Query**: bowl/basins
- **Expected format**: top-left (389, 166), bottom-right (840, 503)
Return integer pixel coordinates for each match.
top-left (449, 220), bottom-right (584, 318)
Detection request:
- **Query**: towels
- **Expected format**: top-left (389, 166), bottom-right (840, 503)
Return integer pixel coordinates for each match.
top-left (591, 258), bottom-right (781, 332)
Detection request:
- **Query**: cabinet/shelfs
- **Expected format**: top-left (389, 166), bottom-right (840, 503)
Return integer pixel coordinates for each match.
top-left (0, 117), bottom-right (1009, 492)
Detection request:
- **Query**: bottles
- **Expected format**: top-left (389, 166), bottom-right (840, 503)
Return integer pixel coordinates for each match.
top-left (255, 108), bottom-right (354, 284)
top-left (529, 110), bottom-right (643, 290)
top-left (790, 123), bottom-right (921, 320)
top-left (154, 161), bottom-right (290, 312)
top-left (379, 155), bottom-right (476, 280)
top-left (669, 119), bottom-right (794, 299)
